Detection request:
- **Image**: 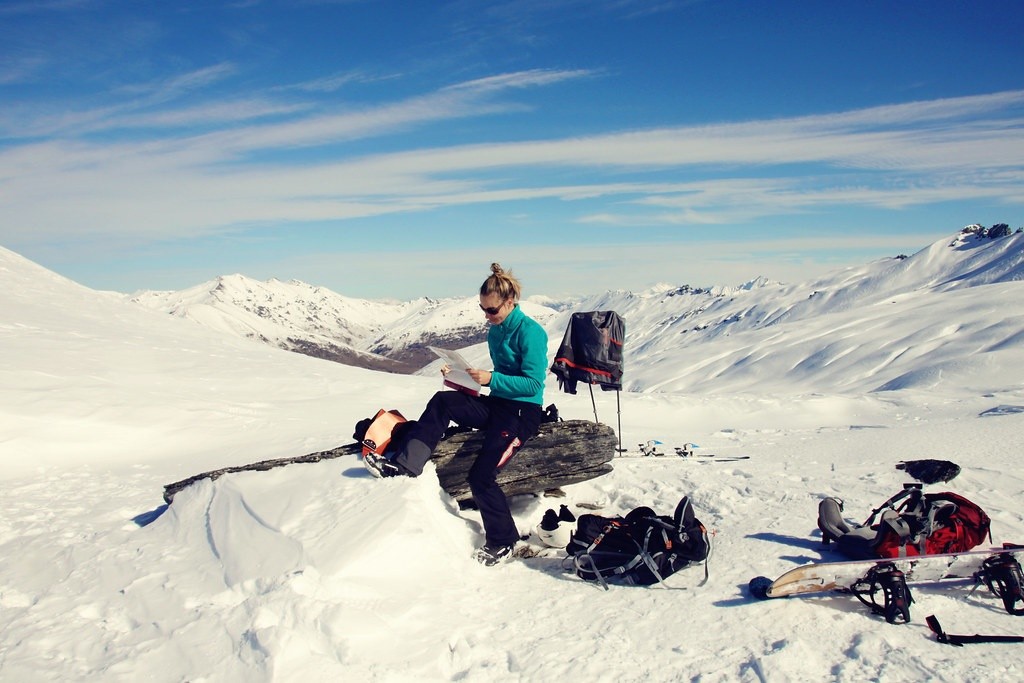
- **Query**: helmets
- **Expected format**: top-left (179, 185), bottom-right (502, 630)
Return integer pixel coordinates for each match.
top-left (537, 504), bottom-right (576, 548)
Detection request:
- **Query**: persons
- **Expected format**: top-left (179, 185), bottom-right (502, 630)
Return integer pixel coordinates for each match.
top-left (364, 263), bottom-right (549, 567)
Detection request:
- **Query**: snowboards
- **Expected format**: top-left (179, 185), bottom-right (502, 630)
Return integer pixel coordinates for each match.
top-left (766, 548), bottom-right (1024, 600)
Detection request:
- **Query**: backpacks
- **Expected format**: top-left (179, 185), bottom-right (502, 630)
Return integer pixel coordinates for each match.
top-left (816, 483), bottom-right (992, 559)
top-left (565, 496), bottom-right (713, 592)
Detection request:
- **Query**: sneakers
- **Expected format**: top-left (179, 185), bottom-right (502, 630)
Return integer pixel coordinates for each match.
top-left (364, 452), bottom-right (417, 479)
top-left (472, 538), bottom-right (530, 567)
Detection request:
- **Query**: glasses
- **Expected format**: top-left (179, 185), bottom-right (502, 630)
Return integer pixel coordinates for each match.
top-left (479, 298), bottom-right (507, 315)
